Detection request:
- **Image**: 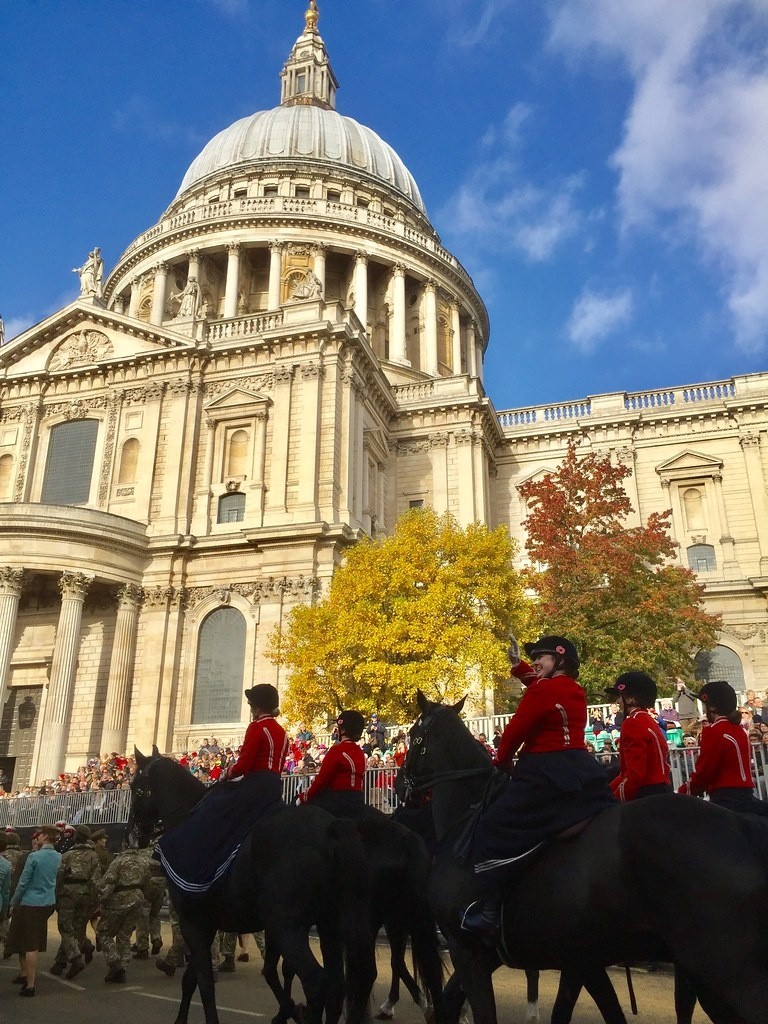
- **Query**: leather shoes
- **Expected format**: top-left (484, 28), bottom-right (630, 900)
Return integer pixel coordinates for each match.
top-left (18, 987), bottom-right (35, 997)
top-left (11, 976), bottom-right (27, 984)
top-left (450, 906), bottom-right (502, 947)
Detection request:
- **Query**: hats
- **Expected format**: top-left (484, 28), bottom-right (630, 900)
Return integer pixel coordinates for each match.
top-left (64, 826), bottom-right (75, 834)
top-left (37, 825), bottom-right (62, 835)
top-left (91, 829), bottom-right (109, 838)
top-left (76, 825), bottom-right (91, 839)
top-left (5, 826), bottom-right (16, 832)
top-left (31, 831), bottom-right (39, 837)
top-left (55, 821), bottom-right (65, 827)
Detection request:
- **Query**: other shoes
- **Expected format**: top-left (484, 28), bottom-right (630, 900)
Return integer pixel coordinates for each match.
top-left (49, 963), bottom-right (63, 975)
top-left (105, 963), bottom-right (126, 983)
top-left (133, 949), bottom-right (149, 959)
top-left (237, 953), bottom-right (249, 962)
top-left (130, 944), bottom-right (138, 952)
top-left (151, 938), bottom-right (163, 954)
top-left (84, 942), bottom-right (95, 964)
top-left (155, 959), bottom-right (176, 977)
top-left (66, 955), bottom-right (87, 978)
top-left (218, 956), bottom-right (235, 972)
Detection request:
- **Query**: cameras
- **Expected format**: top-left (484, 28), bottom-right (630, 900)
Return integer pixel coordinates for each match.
top-left (681, 687), bottom-right (684, 692)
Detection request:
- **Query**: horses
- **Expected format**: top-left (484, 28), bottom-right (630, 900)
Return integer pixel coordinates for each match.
top-left (123, 686), bottom-right (768, 1024)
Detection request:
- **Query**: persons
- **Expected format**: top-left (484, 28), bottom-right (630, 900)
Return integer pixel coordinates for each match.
top-left (292, 268), bottom-right (323, 298)
top-left (288, 711), bottom-right (365, 818)
top-left (608, 672), bottom-right (673, 803)
top-left (678, 681), bottom-right (755, 816)
top-left (153, 682), bottom-right (289, 893)
top-left (70, 246), bottom-right (105, 299)
top-left (458, 633), bottom-right (610, 931)
top-left (0, 820), bottom-right (265, 996)
top-left (173, 275), bottom-right (202, 317)
top-left (0, 676), bottom-right (768, 825)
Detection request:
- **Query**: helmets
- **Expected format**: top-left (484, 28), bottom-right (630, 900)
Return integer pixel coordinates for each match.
top-left (604, 672), bottom-right (658, 700)
top-left (337, 710), bottom-right (364, 734)
top-left (524, 636), bottom-right (580, 670)
top-left (245, 684), bottom-right (279, 709)
top-left (690, 681), bottom-right (737, 713)
